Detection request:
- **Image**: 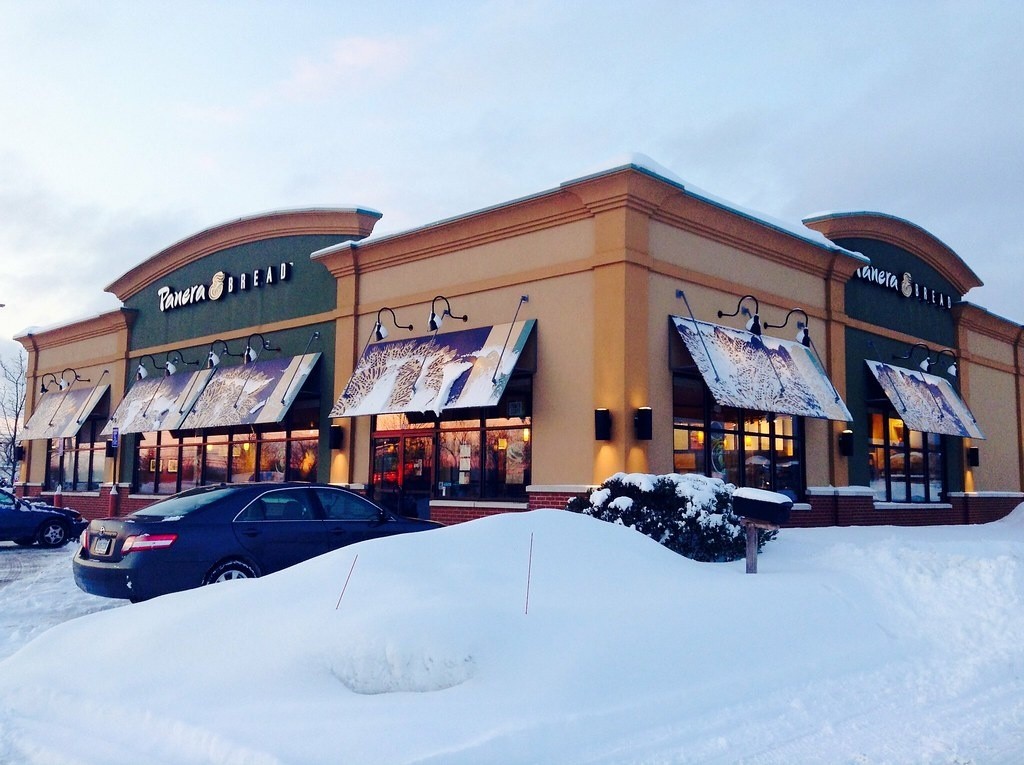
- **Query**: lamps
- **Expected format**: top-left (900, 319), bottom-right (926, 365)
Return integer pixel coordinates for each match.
top-left (892, 343), bottom-right (932, 373)
top-left (427, 296), bottom-right (467, 332)
top-left (40, 373), bottom-right (58, 394)
top-left (718, 295), bottom-right (763, 336)
top-left (244, 333), bottom-right (281, 364)
top-left (967, 446), bottom-right (979, 466)
top-left (329, 425), bottom-right (343, 449)
top-left (373, 307), bottom-right (413, 342)
top-left (106, 438), bottom-right (114, 458)
top-left (635, 406), bottom-right (652, 440)
top-left (164, 349), bottom-right (200, 376)
top-left (764, 308), bottom-right (811, 347)
top-left (136, 355), bottom-right (166, 381)
top-left (839, 429), bottom-right (853, 456)
top-left (595, 408), bottom-right (611, 440)
top-left (16, 445), bottom-right (25, 460)
top-left (206, 339), bottom-right (244, 369)
top-left (60, 368), bottom-right (90, 391)
top-left (930, 350), bottom-right (959, 377)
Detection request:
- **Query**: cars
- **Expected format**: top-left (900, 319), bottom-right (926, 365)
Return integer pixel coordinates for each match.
top-left (880, 451), bottom-right (928, 483)
top-left (720, 456), bottom-right (799, 490)
top-left (0, 488), bottom-right (89, 548)
top-left (72, 481), bottom-right (446, 604)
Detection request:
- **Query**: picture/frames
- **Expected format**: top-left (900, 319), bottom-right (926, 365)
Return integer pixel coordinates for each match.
top-left (149, 458), bottom-right (164, 472)
top-left (167, 459), bottom-right (178, 473)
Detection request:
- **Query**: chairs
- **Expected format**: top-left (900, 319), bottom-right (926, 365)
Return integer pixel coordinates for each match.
top-left (278, 500), bottom-right (303, 520)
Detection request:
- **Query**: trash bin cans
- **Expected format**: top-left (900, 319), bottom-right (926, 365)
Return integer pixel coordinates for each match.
top-left (373, 487), bottom-right (431, 519)
